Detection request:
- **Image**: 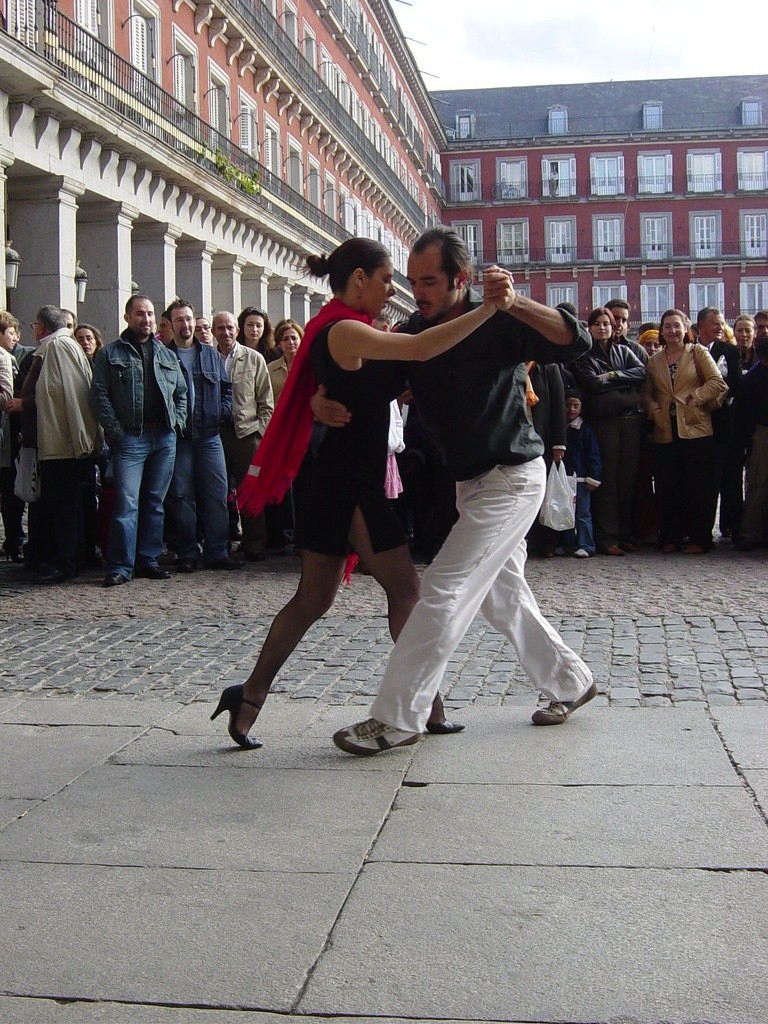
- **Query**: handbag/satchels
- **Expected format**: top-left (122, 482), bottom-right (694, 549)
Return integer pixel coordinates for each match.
top-left (539, 459), bottom-right (577, 531)
top-left (693, 343), bottom-right (730, 411)
top-left (13, 446), bottom-right (41, 503)
top-left (581, 381), bottom-right (642, 420)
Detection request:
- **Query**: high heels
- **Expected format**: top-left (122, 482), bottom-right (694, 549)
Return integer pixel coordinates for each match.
top-left (210, 684), bottom-right (264, 748)
top-left (426, 693), bottom-right (466, 734)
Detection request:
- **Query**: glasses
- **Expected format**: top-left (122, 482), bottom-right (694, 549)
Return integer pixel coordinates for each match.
top-left (30, 323), bottom-right (40, 330)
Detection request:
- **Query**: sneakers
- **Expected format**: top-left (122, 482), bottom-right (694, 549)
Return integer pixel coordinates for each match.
top-left (333, 717), bottom-right (423, 754)
top-left (532, 677), bottom-right (598, 725)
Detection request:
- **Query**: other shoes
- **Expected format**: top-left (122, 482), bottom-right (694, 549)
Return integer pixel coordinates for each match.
top-left (237, 544), bottom-right (266, 561)
top-left (3, 543), bottom-right (25, 563)
top-left (537, 527), bottom-right (768, 558)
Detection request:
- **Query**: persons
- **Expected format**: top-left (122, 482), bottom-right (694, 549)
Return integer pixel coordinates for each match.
top-left (333, 226), bottom-right (593, 757)
top-left (268, 323), bottom-right (305, 554)
top-left (209, 239), bottom-right (514, 750)
top-left (642, 308), bottom-right (725, 553)
top-left (0, 297), bottom-right (767, 584)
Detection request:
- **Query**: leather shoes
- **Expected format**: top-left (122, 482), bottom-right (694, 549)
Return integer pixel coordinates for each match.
top-left (135, 565), bottom-right (171, 579)
top-left (33, 564), bottom-right (80, 584)
top-left (209, 556), bottom-right (244, 570)
top-left (105, 573), bottom-right (125, 584)
top-left (178, 559), bottom-right (197, 572)
